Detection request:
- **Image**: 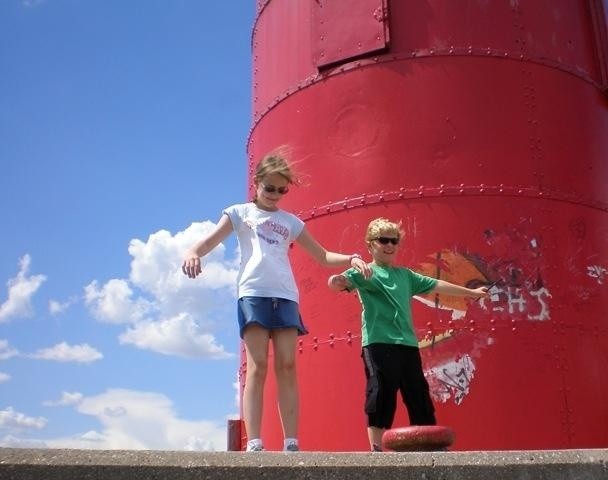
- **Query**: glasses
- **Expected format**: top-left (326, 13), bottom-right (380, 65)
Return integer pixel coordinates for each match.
top-left (259, 181), bottom-right (289, 194)
top-left (370, 236), bottom-right (399, 245)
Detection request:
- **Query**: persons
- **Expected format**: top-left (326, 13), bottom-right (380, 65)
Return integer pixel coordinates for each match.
top-left (179, 147), bottom-right (373, 454)
top-left (326, 218), bottom-right (493, 454)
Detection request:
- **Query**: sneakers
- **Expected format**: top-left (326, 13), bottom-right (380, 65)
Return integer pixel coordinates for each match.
top-left (282, 443), bottom-right (300, 451)
top-left (246, 444), bottom-right (265, 451)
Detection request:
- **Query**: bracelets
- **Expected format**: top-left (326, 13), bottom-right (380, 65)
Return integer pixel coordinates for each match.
top-left (349, 252), bottom-right (362, 268)
